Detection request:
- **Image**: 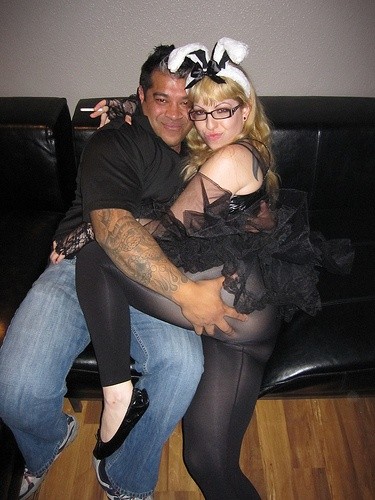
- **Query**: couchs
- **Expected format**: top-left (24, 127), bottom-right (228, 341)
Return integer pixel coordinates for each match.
top-left (0, 95), bottom-right (374, 413)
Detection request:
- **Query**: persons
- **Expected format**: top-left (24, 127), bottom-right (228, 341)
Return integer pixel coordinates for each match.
top-left (0, 45), bottom-right (249, 500)
top-left (49, 36), bottom-right (323, 500)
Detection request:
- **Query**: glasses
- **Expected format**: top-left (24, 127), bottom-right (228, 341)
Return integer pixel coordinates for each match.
top-left (189, 103), bottom-right (241, 121)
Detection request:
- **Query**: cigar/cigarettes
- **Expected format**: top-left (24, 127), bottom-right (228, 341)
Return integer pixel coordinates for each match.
top-left (80, 108), bottom-right (104, 111)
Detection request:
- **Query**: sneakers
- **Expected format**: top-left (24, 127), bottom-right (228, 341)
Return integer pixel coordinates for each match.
top-left (91, 454), bottom-right (154, 500)
top-left (19, 414), bottom-right (78, 500)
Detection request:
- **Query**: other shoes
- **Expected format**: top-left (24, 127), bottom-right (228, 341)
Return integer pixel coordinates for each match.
top-left (93, 389), bottom-right (149, 459)
